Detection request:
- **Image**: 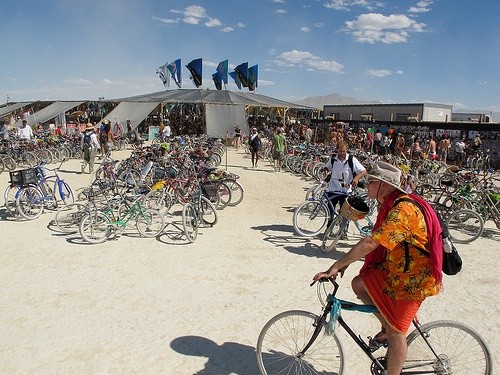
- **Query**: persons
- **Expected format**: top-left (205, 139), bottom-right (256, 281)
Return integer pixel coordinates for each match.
top-left (126, 119), bottom-right (142, 145)
top-left (249, 128), bottom-right (261, 168)
top-left (99, 119), bottom-right (107, 159)
top-left (163, 123), bottom-right (170, 143)
top-left (72, 127), bottom-right (82, 138)
top-left (270, 129), bottom-right (288, 173)
top-left (16, 119), bottom-right (34, 161)
top-left (314, 162), bottom-right (445, 375)
top-left (323, 140), bottom-right (367, 239)
top-left (81, 123), bottom-right (101, 174)
top-left (102, 124), bottom-right (115, 165)
top-left (56, 125), bottom-right (63, 136)
top-left (234, 125), bottom-right (241, 151)
top-left (114, 121), bottom-right (124, 141)
top-left (247, 116), bottom-right (483, 168)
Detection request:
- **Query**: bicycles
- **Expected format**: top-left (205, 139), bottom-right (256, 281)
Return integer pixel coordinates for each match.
top-left (255, 268), bottom-right (494, 375)
top-left (0, 130), bottom-right (244, 244)
top-left (239, 131), bottom-right (500, 253)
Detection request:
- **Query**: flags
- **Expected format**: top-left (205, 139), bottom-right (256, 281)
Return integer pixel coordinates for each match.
top-left (156, 58), bottom-right (260, 90)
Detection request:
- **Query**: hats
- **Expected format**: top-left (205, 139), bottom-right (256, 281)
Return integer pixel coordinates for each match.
top-left (85, 123), bottom-right (94, 130)
top-left (369, 161), bottom-right (409, 195)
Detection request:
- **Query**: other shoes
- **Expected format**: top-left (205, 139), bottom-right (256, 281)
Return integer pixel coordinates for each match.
top-left (324, 228), bottom-right (332, 235)
top-left (82, 165), bottom-right (85, 173)
top-left (89, 171), bottom-right (94, 174)
top-left (341, 234), bottom-right (348, 241)
top-left (369, 328), bottom-right (387, 347)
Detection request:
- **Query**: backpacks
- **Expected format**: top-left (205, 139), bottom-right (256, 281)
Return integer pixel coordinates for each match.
top-left (390, 197), bottom-right (463, 276)
top-left (100, 132), bottom-right (108, 142)
top-left (83, 132), bottom-right (94, 149)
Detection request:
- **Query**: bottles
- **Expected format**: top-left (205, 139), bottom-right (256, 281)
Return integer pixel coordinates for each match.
top-left (344, 171), bottom-right (350, 192)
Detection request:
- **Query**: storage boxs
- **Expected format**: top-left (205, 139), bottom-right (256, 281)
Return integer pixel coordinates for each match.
top-left (8, 167), bottom-right (38, 185)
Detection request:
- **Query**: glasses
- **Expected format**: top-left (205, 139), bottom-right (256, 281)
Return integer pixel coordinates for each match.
top-left (367, 178), bottom-right (379, 184)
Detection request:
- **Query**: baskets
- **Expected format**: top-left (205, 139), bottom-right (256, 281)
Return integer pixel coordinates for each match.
top-left (340, 197), bottom-right (370, 221)
top-left (203, 181), bottom-right (218, 196)
